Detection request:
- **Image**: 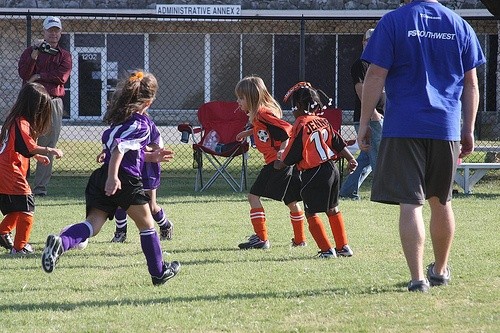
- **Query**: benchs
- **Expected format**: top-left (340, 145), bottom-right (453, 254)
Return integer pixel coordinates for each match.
top-left (456, 146), bottom-right (500, 195)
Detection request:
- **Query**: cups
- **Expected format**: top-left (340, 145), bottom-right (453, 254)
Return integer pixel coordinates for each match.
top-left (181, 123), bottom-right (192, 143)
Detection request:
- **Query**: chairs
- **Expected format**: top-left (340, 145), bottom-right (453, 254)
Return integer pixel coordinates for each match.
top-left (315, 108), bottom-right (357, 190)
top-left (178, 100), bottom-right (254, 194)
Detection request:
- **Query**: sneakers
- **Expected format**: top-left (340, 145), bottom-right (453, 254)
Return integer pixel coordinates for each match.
top-left (408, 280), bottom-right (429, 292)
top-left (315, 248), bottom-right (336, 258)
top-left (0, 234), bottom-right (15, 249)
top-left (12, 244), bottom-right (33, 254)
top-left (427, 263), bottom-right (450, 286)
top-left (160, 221), bottom-right (174, 240)
top-left (335, 245), bottom-right (353, 257)
top-left (151, 260), bottom-right (181, 286)
top-left (292, 238), bottom-right (307, 247)
top-left (111, 232), bottom-right (125, 243)
top-left (238, 235), bottom-right (269, 249)
top-left (42, 235), bottom-right (65, 273)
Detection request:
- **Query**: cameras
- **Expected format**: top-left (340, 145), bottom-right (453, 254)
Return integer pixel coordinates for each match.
top-left (41, 41), bottom-right (58, 57)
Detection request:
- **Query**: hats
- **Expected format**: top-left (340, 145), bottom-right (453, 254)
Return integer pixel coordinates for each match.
top-left (43, 17), bottom-right (62, 30)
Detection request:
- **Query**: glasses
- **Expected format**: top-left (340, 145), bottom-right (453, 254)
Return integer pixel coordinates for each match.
top-left (46, 28), bottom-right (60, 33)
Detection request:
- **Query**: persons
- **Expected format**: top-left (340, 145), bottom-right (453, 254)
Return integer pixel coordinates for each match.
top-left (356, 0), bottom-right (487, 293)
top-left (338, 28), bottom-right (387, 200)
top-left (0, 82), bottom-right (63, 255)
top-left (18, 16), bottom-right (72, 197)
top-left (235, 77), bottom-right (307, 249)
top-left (98, 110), bottom-right (174, 243)
top-left (282, 86), bottom-right (358, 258)
top-left (42, 71), bottom-right (181, 286)
